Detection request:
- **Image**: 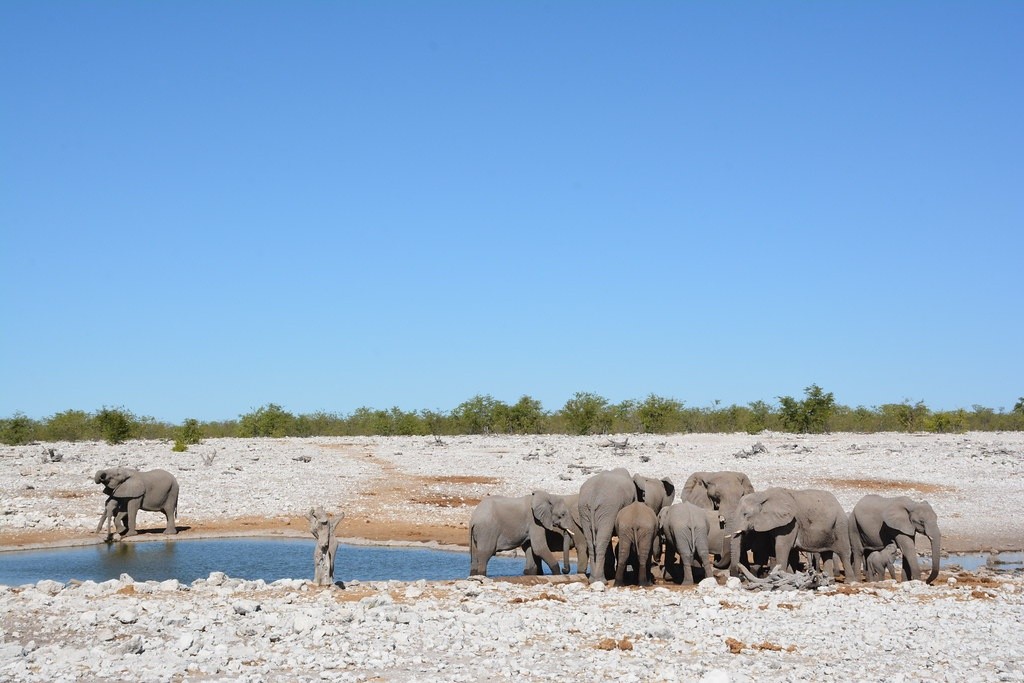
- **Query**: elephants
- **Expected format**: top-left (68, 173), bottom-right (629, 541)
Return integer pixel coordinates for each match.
top-left (466, 468), bottom-right (941, 582)
top-left (94, 467), bottom-right (179, 536)
